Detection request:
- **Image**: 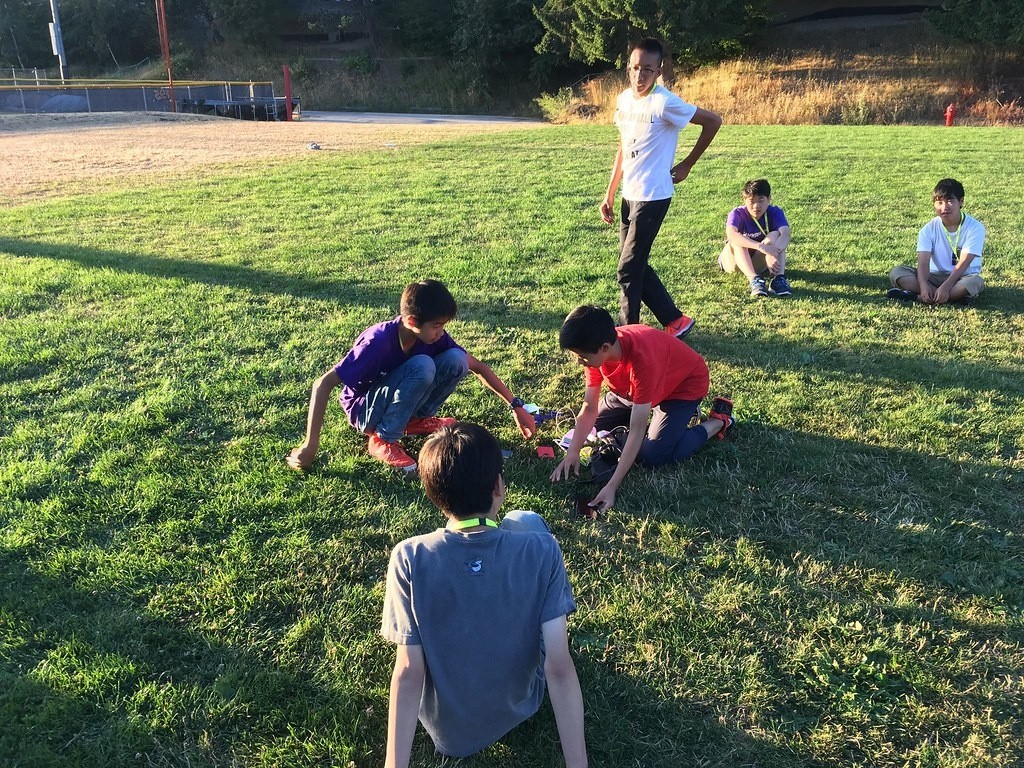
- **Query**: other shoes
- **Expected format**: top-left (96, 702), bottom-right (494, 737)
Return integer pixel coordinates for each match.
top-left (887, 287), bottom-right (919, 302)
top-left (959, 296), bottom-right (972, 307)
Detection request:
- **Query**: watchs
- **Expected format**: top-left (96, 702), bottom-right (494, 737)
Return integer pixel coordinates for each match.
top-left (508, 396), bottom-right (525, 410)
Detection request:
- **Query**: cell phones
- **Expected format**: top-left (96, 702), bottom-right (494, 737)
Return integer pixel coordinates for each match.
top-left (575, 499), bottom-right (598, 518)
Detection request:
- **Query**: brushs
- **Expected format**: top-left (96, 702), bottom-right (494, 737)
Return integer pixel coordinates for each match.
top-left (576, 499), bottom-right (600, 521)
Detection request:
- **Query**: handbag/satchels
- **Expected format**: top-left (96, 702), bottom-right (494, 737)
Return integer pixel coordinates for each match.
top-left (573, 425), bottom-right (629, 518)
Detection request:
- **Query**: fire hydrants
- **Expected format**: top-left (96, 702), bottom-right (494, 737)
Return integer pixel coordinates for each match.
top-left (943, 101), bottom-right (956, 127)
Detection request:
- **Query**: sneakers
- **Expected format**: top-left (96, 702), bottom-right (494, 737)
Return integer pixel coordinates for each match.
top-left (665, 314), bottom-right (696, 340)
top-left (768, 273), bottom-right (791, 296)
top-left (751, 275), bottom-right (768, 297)
top-left (709, 397), bottom-right (737, 440)
top-left (368, 430), bottom-right (417, 472)
top-left (692, 405), bottom-right (702, 420)
top-left (403, 416), bottom-right (457, 435)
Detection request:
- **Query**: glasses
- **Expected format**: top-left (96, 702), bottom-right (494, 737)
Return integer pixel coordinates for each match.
top-left (627, 63), bottom-right (660, 76)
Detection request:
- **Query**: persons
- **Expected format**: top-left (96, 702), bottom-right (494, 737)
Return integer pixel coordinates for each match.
top-left (599, 36), bottom-right (723, 339)
top-left (717, 179), bottom-right (792, 297)
top-left (549, 305), bottom-right (736, 511)
top-left (887, 179), bottom-right (986, 306)
top-left (287, 279), bottom-right (536, 474)
top-left (375, 422), bottom-right (590, 768)
